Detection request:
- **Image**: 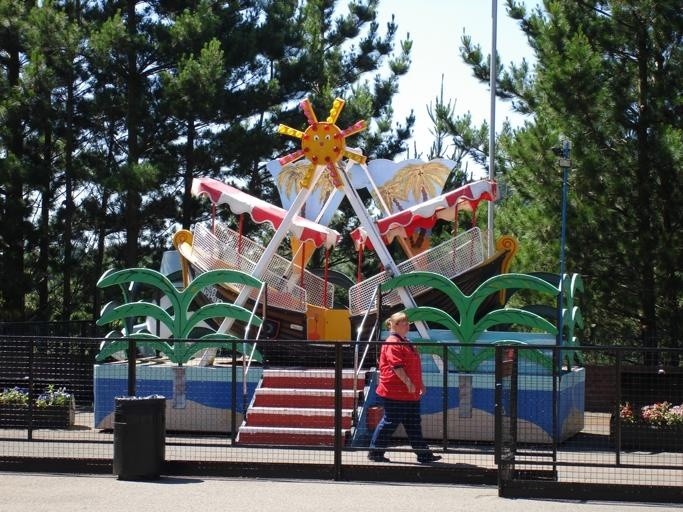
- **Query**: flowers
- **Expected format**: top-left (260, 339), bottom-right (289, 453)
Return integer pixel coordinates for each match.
top-left (611, 399), bottom-right (682, 427)
top-left (0, 384), bottom-right (75, 409)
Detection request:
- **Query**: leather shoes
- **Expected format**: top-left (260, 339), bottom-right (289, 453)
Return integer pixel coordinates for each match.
top-left (417, 452), bottom-right (442, 462)
top-left (367, 452), bottom-right (390, 462)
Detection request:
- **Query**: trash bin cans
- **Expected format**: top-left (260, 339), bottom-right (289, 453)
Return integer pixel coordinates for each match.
top-left (114, 396), bottom-right (166, 480)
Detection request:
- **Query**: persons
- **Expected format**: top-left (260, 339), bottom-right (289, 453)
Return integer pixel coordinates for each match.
top-left (369, 312), bottom-right (442, 462)
